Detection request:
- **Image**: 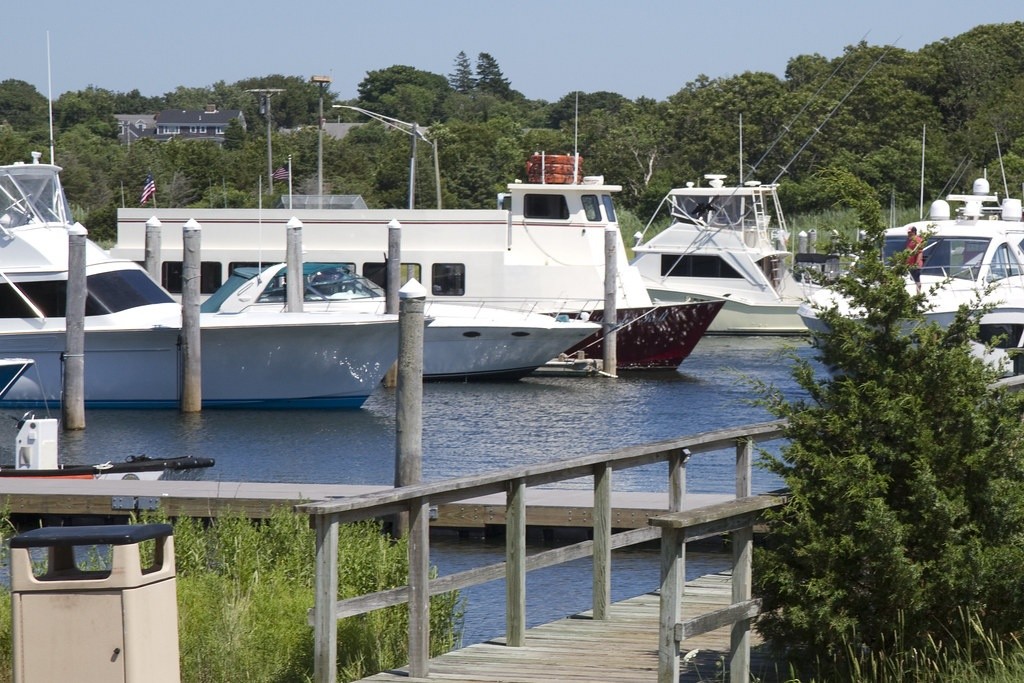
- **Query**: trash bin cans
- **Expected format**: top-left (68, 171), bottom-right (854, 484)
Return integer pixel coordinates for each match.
top-left (11, 523), bottom-right (180, 683)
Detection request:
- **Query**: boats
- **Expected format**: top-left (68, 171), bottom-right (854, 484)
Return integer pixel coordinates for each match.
top-left (796, 177), bottom-right (1024, 340)
top-left (200, 262), bottom-right (604, 384)
top-left (1, 152), bottom-right (434, 416)
top-left (536, 358), bottom-right (602, 375)
top-left (104, 154), bottom-right (732, 371)
top-left (947, 274), bottom-right (1024, 393)
top-left (628, 172), bottom-right (823, 332)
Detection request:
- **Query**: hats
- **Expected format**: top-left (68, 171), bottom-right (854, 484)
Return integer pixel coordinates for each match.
top-left (907, 226), bottom-right (917, 233)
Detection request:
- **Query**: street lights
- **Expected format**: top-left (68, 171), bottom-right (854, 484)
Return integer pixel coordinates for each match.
top-left (332, 104), bottom-right (419, 210)
top-left (413, 130), bottom-right (441, 210)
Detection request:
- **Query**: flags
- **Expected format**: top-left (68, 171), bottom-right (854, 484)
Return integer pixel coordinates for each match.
top-left (272, 162), bottom-right (290, 182)
top-left (140, 172), bottom-right (155, 205)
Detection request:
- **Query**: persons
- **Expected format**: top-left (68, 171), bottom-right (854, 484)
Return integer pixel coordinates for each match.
top-left (905, 226), bottom-right (923, 296)
